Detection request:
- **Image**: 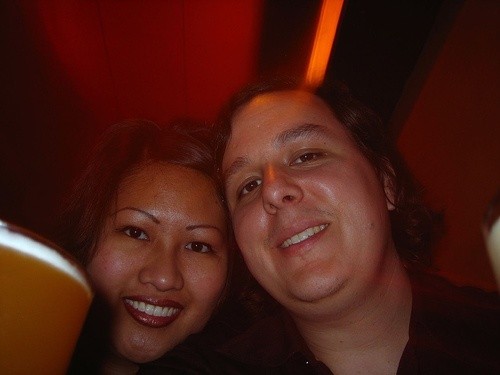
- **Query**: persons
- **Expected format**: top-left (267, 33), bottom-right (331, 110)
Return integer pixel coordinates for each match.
top-left (47, 117), bottom-right (256, 375)
top-left (179, 69), bottom-right (500, 375)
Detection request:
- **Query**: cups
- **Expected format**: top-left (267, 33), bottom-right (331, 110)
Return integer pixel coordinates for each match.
top-left (1, 221), bottom-right (97, 375)
